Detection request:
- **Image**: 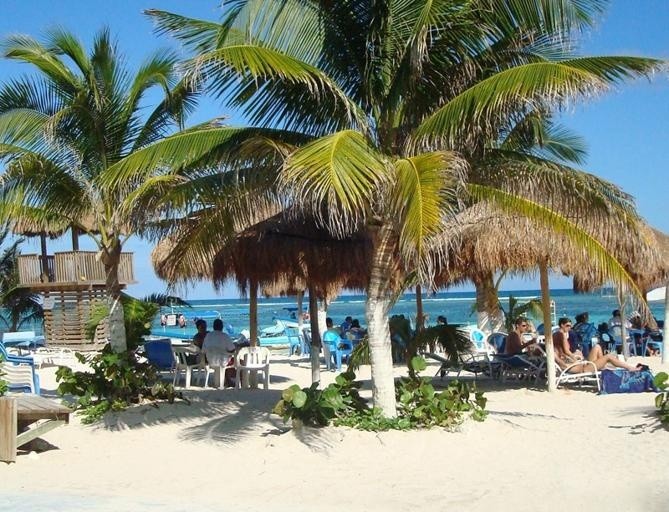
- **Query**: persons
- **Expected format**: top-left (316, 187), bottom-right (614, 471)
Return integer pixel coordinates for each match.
top-left (500, 306), bottom-right (659, 375)
top-left (202, 320), bottom-right (238, 384)
top-left (324, 313), bottom-right (363, 367)
top-left (194, 319), bottom-right (209, 348)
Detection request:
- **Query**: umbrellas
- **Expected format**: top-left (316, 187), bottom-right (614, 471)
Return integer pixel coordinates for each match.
top-left (644, 286), bottom-right (666, 301)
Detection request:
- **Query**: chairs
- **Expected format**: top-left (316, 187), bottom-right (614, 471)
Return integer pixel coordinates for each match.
top-left (0, 316), bottom-right (664, 396)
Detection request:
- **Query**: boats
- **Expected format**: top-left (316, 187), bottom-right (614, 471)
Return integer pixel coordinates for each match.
top-left (256, 306), bottom-right (342, 347)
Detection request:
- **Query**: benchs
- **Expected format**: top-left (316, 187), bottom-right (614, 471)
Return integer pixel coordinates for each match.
top-left (0, 394), bottom-right (73, 462)
